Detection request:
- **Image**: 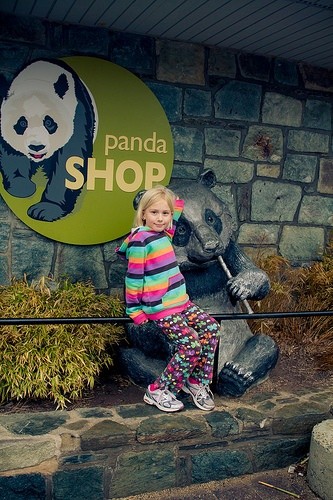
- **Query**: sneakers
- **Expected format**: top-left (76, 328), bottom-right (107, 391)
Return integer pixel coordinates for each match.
top-left (182, 380), bottom-right (216, 413)
top-left (142, 385), bottom-right (185, 413)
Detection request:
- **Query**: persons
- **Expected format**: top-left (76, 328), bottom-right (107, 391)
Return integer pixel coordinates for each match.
top-left (115, 185), bottom-right (222, 413)
